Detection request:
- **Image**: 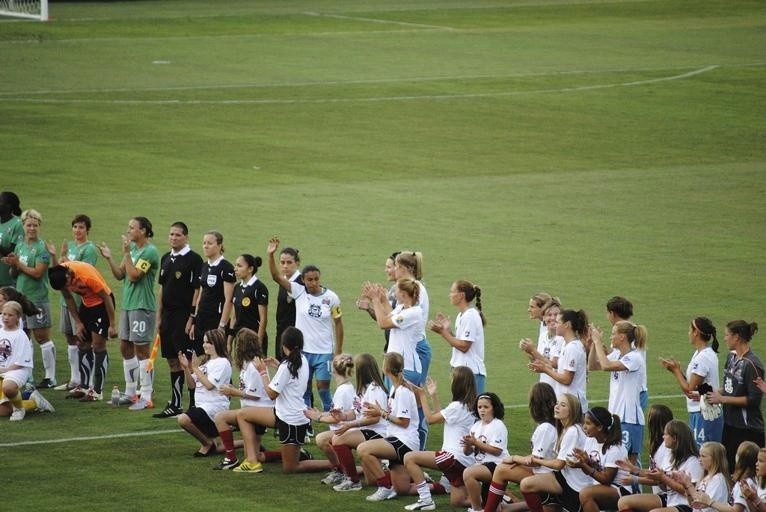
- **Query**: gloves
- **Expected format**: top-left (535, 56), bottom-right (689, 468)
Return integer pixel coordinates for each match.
top-left (697, 383), bottom-right (722, 421)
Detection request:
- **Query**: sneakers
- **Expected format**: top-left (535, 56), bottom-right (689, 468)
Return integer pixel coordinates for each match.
top-left (213, 444), bottom-right (265, 473)
top-left (128, 400), bottom-right (154, 410)
top-left (321, 458), bottom-right (390, 491)
top-left (39, 379), bottom-right (103, 402)
top-left (153, 401), bottom-right (184, 418)
top-left (366, 485), bottom-right (397, 501)
top-left (106, 395), bottom-right (138, 405)
top-left (193, 439), bottom-right (216, 457)
top-left (10, 383), bottom-right (56, 421)
top-left (299, 423), bottom-right (314, 461)
top-left (404, 472), bottom-right (451, 511)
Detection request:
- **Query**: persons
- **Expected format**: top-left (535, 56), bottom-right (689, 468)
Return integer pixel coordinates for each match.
top-left (519, 292), bottom-right (766, 512)
top-left (356, 251), bottom-right (487, 396)
top-left (97, 217), bottom-right (343, 472)
top-left (1, 189), bottom-right (116, 423)
top-left (303, 351), bottom-right (528, 512)
